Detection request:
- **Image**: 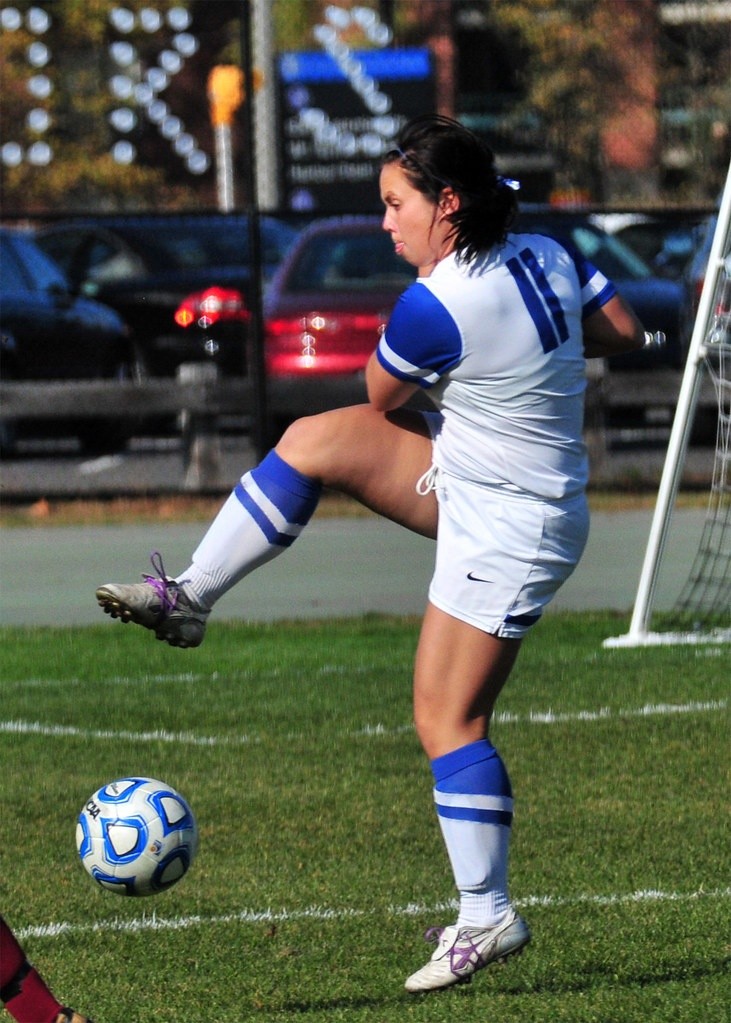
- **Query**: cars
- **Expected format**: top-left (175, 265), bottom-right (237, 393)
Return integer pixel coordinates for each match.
top-left (678, 212), bottom-right (731, 395)
top-left (30, 213), bottom-right (318, 443)
top-left (0, 229), bottom-right (140, 466)
top-left (253, 212), bottom-right (448, 478)
top-left (511, 202), bottom-right (690, 372)
top-left (588, 212), bottom-right (695, 282)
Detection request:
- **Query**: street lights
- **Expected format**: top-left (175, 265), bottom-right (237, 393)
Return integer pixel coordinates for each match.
top-left (209, 65), bottom-right (245, 217)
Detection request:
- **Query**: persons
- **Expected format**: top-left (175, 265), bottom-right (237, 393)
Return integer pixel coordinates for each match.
top-left (96, 111), bottom-right (597, 993)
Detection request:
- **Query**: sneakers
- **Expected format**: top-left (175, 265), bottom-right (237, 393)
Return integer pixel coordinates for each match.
top-left (93, 576), bottom-right (212, 650)
top-left (403, 907), bottom-right (531, 994)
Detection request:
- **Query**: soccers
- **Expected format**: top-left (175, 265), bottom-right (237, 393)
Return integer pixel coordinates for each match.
top-left (74, 776), bottom-right (194, 896)
